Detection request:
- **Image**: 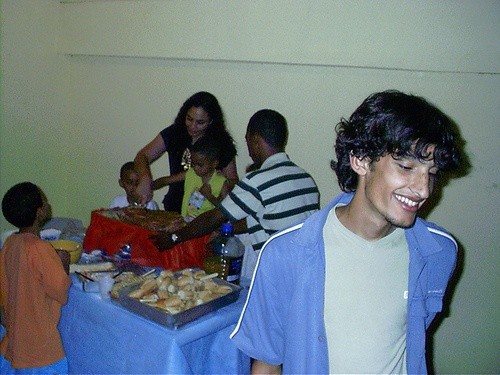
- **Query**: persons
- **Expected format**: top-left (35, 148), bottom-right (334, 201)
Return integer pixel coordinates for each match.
top-left (109, 161), bottom-right (160, 210)
top-left (228, 88), bottom-right (458, 375)
top-left (151, 140), bottom-right (229, 223)
top-left (134, 91), bottom-right (239, 212)
top-left (0, 181), bottom-right (73, 375)
top-left (148, 109), bottom-right (320, 281)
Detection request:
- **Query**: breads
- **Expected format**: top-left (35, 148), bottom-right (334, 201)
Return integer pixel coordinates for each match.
top-left (112, 268), bottom-right (231, 314)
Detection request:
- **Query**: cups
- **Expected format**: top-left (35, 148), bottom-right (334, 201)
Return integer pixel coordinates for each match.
top-left (99, 278), bottom-right (114, 298)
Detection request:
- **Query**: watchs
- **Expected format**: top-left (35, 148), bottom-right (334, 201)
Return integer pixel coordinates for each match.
top-left (171, 234), bottom-right (179, 242)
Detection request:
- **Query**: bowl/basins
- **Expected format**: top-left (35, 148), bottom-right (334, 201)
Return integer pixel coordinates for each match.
top-left (49, 240), bottom-right (83, 264)
top-left (40, 229), bottom-right (61, 241)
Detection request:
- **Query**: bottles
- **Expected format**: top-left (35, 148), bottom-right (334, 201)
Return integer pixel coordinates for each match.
top-left (204, 224), bottom-right (245, 286)
